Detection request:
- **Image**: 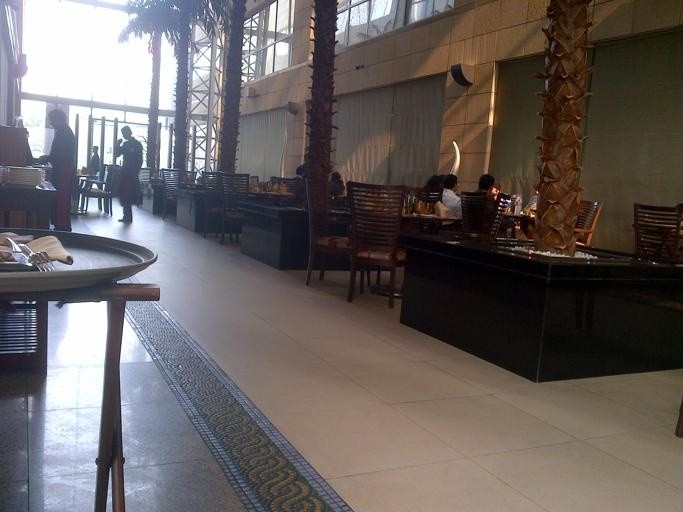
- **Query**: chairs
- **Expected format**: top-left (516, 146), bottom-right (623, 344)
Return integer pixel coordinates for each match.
top-left (633, 203), bottom-right (683, 264)
top-left (573, 199), bottom-right (604, 247)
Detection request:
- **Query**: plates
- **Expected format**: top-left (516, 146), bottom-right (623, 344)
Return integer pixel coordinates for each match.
top-left (8, 166), bottom-right (42, 185)
top-left (0, 259), bottom-right (37, 272)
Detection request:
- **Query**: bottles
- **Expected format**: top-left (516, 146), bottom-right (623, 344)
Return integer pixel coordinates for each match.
top-left (514, 193), bottom-right (522, 216)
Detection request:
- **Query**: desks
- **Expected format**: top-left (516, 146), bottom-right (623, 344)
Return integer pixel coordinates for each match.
top-left (1, 179), bottom-right (57, 230)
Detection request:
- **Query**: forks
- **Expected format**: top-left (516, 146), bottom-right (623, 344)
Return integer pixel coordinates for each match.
top-left (4, 232), bottom-right (33, 266)
top-left (18, 242), bottom-right (55, 272)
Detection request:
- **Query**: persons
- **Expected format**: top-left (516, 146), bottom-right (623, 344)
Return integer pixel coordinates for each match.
top-left (82, 145), bottom-right (100, 189)
top-left (329, 171), bottom-right (346, 197)
top-left (423, 172), bottom-right (540, 233)
top-left (295, 164), bottom-right (305, 181)
top-left (116, 126), bottom-right (143, 223)
top-left (41, 108), bottom-right (75, 231)
top-left (23, 126), bottom-right (48, 167)
